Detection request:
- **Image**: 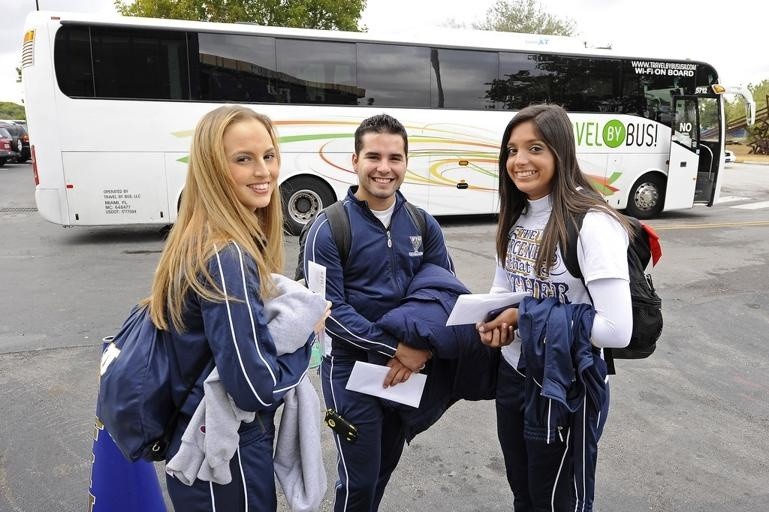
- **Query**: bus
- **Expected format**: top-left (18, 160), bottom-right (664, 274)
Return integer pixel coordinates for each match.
top-left (17, 4), bottom-right (759, 243)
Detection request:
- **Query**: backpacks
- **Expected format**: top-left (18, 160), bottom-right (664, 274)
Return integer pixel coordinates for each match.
top-left (559, 188), bottom-right (663, 360)
top-left (295, 201), bottom-right (427, 281)
top-left (96, 303), bottom-right (211, 461)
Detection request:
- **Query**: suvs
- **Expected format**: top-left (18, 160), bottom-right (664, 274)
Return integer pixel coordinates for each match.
top-left (0, 118), bottom-right (31, 166)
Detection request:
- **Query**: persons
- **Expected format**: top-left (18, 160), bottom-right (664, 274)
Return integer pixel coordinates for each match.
top-left (302, 113), bottom-right (497, 512)
top-left (470, 103), bottom-right (635, 512)
top-left (134, 102), bottom-right (333, 512)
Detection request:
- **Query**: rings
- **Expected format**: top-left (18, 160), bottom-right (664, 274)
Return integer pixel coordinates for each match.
top-left (420, 364), bottom-right (426, 370)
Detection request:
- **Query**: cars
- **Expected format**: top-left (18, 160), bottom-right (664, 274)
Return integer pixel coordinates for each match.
top-left (723, 150), bottom-right (737, 166)
